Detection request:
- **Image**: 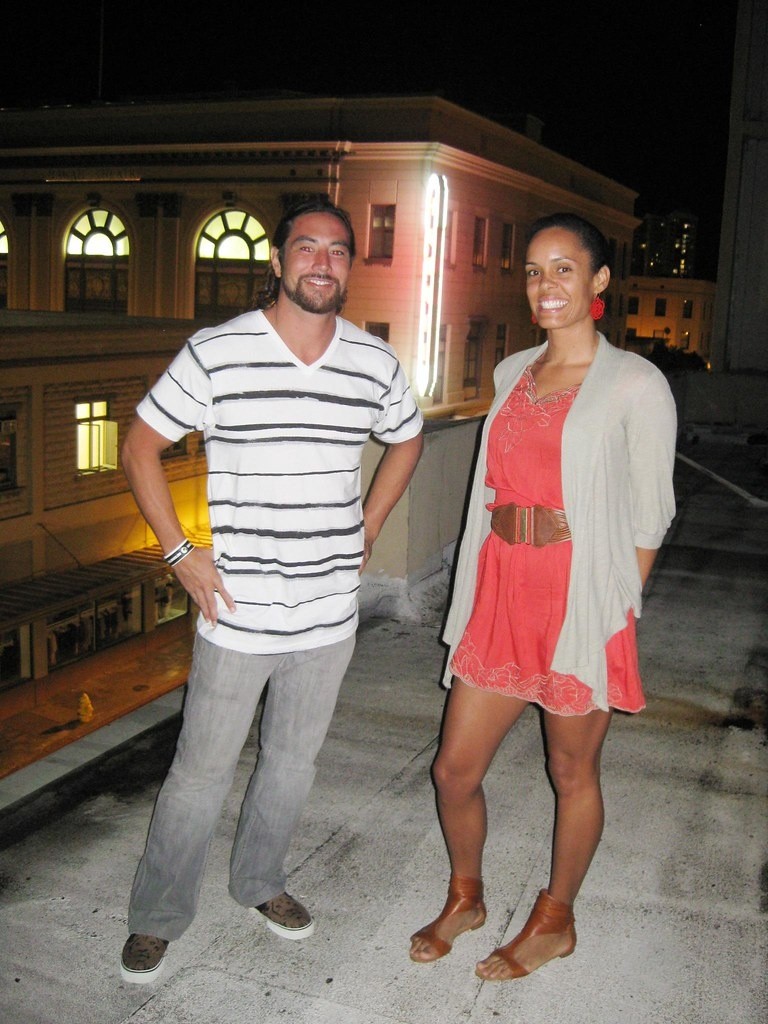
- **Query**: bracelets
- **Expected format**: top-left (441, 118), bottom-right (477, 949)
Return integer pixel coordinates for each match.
top-left (163, 538), bottom-right (195, 568)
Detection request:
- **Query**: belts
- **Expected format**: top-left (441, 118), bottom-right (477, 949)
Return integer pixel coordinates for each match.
top-left (491, 505), bottom-right (571, 548)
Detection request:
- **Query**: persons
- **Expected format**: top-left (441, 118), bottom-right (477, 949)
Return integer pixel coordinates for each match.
top-left (119, 201), bottom-right (425, 983)
top-left (409, 211), bottom-right (677, 982)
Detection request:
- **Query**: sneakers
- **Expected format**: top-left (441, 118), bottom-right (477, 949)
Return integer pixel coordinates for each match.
top-left (118, 934), bottom-right (171, 984)
top-left (251, 890), bottom-right (314, 941)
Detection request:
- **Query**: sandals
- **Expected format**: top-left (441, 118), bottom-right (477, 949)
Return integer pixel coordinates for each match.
top-left (476, 889), bottom-right (576, 980)
top-left (409, 872), bottom-right (486, 962)
